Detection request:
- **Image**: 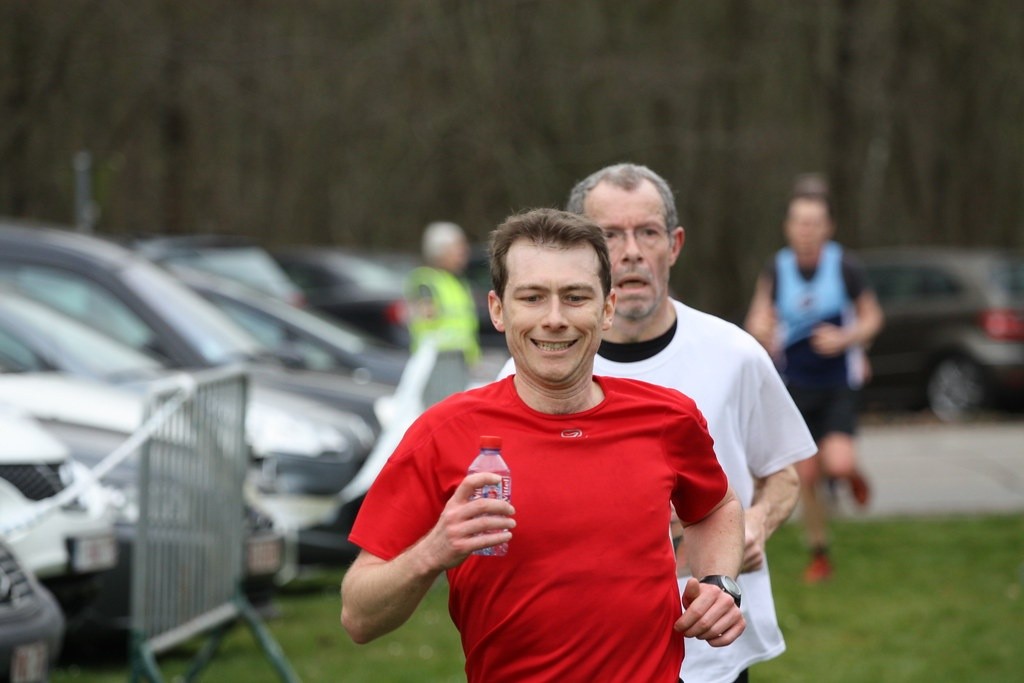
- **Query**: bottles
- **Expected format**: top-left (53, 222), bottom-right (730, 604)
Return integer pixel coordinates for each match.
top-left (468, 434), bottom-right (512, 556)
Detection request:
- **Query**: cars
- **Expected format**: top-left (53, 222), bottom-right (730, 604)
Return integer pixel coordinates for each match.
top-left (0, 215), bottom-right (506, 675)
top-left (831, 240), bottom-right (1024, 431)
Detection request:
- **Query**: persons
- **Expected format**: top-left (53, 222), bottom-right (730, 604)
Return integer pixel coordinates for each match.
top-left (743, 178), bottom-right (886, 584)
top-left (341, 206), bottom-right (745, 683)
top-left (400, 220), bottom-right (484, 408)
top-left (564, 163), bottom-right (818, 683)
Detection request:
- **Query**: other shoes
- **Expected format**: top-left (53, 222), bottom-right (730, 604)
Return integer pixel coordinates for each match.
top-left (805, 557), bottom-right (830, 582)
top-left (848, 474), bottom-right (867, 502)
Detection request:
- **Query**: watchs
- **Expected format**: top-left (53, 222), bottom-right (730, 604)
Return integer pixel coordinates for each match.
top-left (694, 573), bottom-right (742, 608)
